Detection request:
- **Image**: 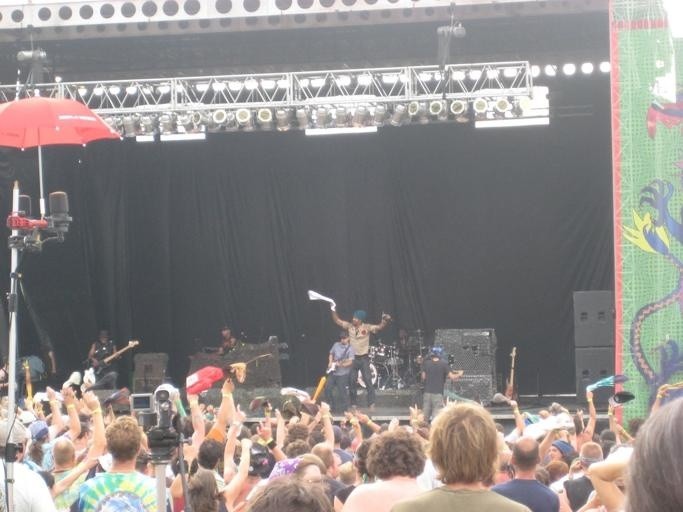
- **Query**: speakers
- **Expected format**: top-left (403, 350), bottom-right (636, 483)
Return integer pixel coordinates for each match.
top-left (573, 290), bottom-right (616, 347)
top-left (575, 347), bottom-right (615, 414)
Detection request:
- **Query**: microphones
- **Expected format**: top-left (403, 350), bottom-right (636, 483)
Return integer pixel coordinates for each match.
top-left (382, 314), bottom-right (393, 323)
top-left (18, 194), bottom-right (32, 219)
top-left (49, 190), bottom-right (69, 243)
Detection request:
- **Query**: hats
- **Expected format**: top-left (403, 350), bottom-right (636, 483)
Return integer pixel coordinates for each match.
top-left (493, 393), bottom-right (509, 405)
top-left (248, 396), bottom-right (319, 416)
top-left (354, 310), bottom-right (366, 320)
top-left (0, 418), bottom-right (30, 447)
top-left (608, 391), bottom-right (634, 407)
top-left (553, 441), bottom-right (575, 458)
top-left (98, 331), bottom-right (110, 338)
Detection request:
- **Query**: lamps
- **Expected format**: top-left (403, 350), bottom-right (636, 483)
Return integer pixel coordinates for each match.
top-left (103, 96), bottom-right (514, 138)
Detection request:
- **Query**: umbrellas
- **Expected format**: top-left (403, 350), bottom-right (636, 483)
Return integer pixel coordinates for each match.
top-left (1, 88), bottom-right (124, 216)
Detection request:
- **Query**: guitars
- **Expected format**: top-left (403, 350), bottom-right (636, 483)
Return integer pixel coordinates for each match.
top-left (89, 340), bottom-right (139, 375)
top-left (326, 342), bottom-right (351, 374)
top-left (505, 346), bottom-right (520, 404)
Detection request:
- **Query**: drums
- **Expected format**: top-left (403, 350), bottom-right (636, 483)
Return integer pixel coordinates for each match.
top-left (369, 346), bottom-right (378, 358)
top-left (358, 362), bottom-right (389, 389)
top-left (377, 344), bottom-right (390, 356)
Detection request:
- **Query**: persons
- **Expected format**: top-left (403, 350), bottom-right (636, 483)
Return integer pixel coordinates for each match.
top-left (323, 331), bottom-right (350, 411)
top-left (0, 366), bottom-right (682, 511)
top-left (421, 345), bottom-right (463, 421)
top-left (217, 326), bottom-right (236, 356)
top-left (330, 303), bottom-right (393, 408)
top-left (88, 331), bottom-right (122, 377)
